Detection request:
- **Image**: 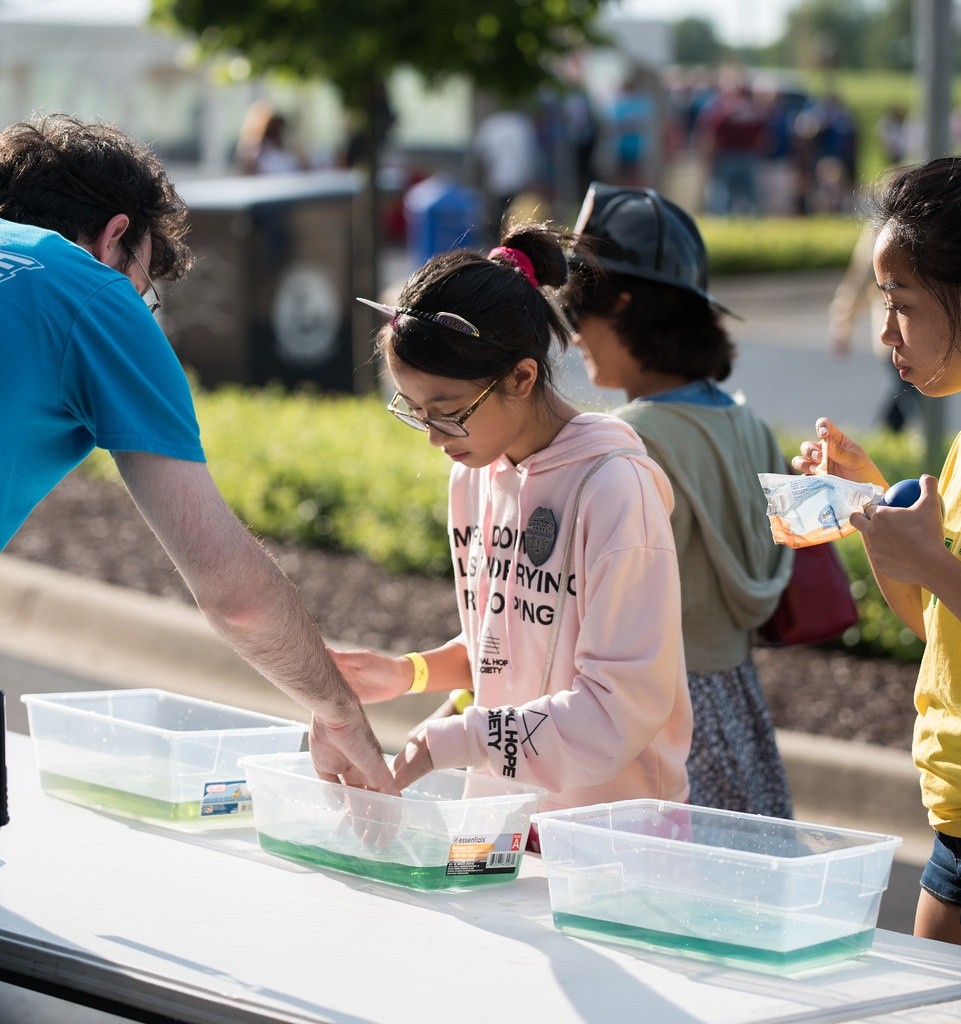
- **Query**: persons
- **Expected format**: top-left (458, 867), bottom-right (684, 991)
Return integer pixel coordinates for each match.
top-left (324, 227), bottom-right (692, 879)
top-left (789, 159), bottom-right (961, 945)
top-left (0, 118), bottom-right (403, 851)
top-left (828, 219), bottom-right (913, 430)
top-left (234, 105), bottom-right (294, 175)
top-left (874, 103), bottom-right (907, 161)
top-left (562, 184), bottom-right (797, 836)
top-left (610, 63), bottom-right (856, 214)
top-left (332, 70), bottom-right (601, 228)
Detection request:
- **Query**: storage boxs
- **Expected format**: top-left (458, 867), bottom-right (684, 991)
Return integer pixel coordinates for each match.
top-left (236, 749), bottom-right (547, 891)
top-left (531, 799), bottom-right (903, 978)
top-left (20, 687), bottom-right (308, 835)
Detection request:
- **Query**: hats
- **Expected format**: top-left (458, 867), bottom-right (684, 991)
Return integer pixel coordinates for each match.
top-left (567, 182), bottom-right (748, 322)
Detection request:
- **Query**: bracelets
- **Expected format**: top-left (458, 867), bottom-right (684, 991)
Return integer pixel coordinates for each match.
top-left (403, 652), bottom-right (428, 695)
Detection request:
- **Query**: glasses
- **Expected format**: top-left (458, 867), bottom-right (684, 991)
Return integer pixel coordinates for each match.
top-left (387, 368), bottom-right (515, 438)
top-left (86, 231), bottom-right (162, 316)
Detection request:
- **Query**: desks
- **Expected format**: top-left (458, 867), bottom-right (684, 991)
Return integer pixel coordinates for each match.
top-left (0, 727), bottom-right (960, 1024)
